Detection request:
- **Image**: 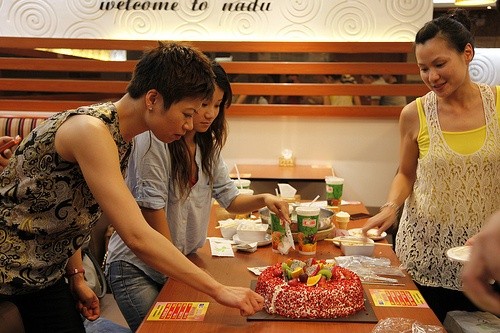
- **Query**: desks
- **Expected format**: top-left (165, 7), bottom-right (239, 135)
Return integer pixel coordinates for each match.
top-left (134, 198), bottom-right (450, 333)
top-left (228, 163), bottom-right (335, 195)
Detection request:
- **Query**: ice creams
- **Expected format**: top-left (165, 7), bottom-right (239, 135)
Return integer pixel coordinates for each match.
top-left (336, 211), bottom-right (350, 229)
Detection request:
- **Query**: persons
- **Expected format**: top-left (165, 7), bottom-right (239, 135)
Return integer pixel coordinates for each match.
top-left (0, 45), bottom-right (263, 333)
top-left (0, 135), bottom-right (22, 174)
top-left (461, 207), bottom-right (500, 314)
top-left (101, 62), bottom-right (292, 333)
top-left (362, 8), bottom-right (500, 324)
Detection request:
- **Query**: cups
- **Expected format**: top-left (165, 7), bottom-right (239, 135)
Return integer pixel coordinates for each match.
top-left (325, 176), bottom-right (345, 207)
top-left (233, 179), bottom-right (251, 190)
top-left (336, 215), bottom-right (350, 229)
top-left (296, 207), bottom-right (320, 256)
top-left (268, 206), bottom-right (293, 253)
top-left (235, 190), bottom-right (254, 220)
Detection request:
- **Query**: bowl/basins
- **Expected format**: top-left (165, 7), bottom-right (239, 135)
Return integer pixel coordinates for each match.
top-left (219, 215), bottom-right (268, 242)
top-left (258, 204), bottom-right (336, 233)
top-left (340, 238), bottom-right (375, 256)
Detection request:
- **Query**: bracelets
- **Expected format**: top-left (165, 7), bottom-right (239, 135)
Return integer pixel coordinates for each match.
top-left (380, 202), bottom-right (400, 221)
top-left (65, 268), bottom-right (85, 278)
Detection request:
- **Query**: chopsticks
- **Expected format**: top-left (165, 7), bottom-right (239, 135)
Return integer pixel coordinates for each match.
top-left (324, 239), bottom-right (393, 247)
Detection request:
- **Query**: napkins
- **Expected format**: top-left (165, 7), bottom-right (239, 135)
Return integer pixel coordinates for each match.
top-left (206, 235), bottom-right (237, 245)
top-left (210, 241), bottom-right (235, 257)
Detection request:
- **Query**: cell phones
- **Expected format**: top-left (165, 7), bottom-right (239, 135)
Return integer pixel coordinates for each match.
top-left (0, 135), bottom-right (21, 152)
top-left (350, 213), bottom-right (369, 220)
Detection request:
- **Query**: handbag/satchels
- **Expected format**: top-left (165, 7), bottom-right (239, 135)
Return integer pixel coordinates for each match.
top-left (81, 247), bottom-right (108, 299)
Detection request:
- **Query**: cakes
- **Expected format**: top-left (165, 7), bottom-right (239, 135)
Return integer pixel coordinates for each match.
top-left (255, 257), bottom-right (366, 319)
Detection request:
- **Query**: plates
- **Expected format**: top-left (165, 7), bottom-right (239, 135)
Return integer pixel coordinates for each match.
top-left (233, 233), bottom-right (272, 246)
top-left (333, 236), bottom-right (375, 246)
top-left (347, 228), bottom-right (387, 240)
top-left (446, 246), bottom-right (471, 264)
top-left (263, 223), bottom-right (335, 242)
